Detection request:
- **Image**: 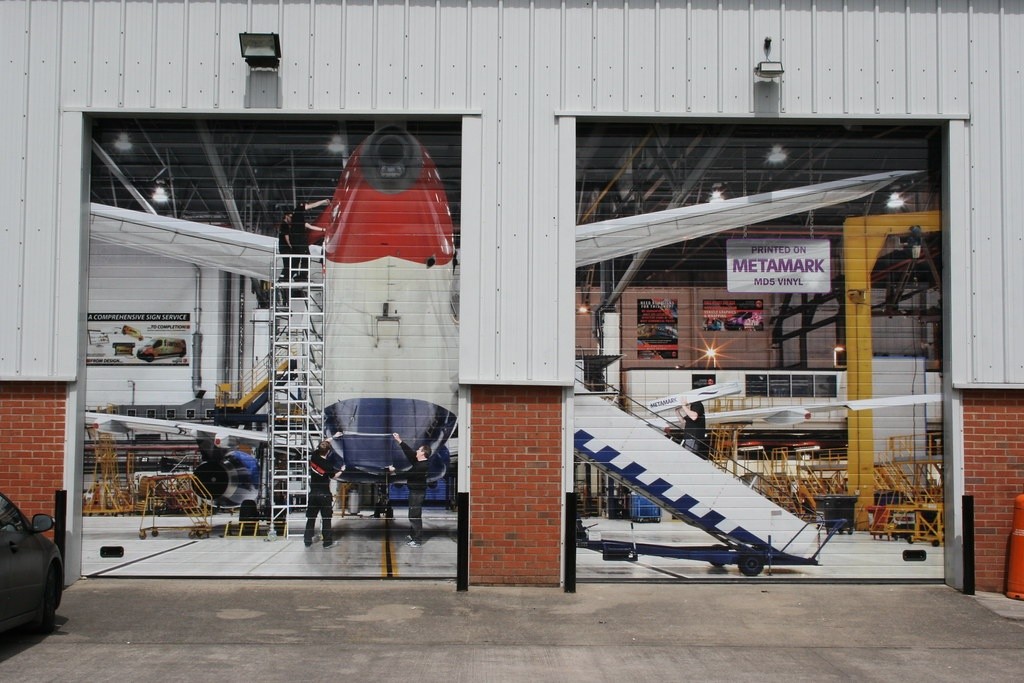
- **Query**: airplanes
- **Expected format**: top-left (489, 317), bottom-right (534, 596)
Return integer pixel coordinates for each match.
top-left (84, 130), bottom-right (931, 566)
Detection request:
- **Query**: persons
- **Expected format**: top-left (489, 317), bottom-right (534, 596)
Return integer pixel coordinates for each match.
top-left (300, 430), bottom-right (349, 549)
top-left (278, 198), bottom-right (332, 284)
top-left (674, 394), bottom-right (705, 455)
top-left (387, 433), bottom-right (434, 547)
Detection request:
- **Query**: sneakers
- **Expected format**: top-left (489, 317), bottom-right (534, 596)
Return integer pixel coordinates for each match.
top-left (404, 540), bottom-right (422, 548)
top-left (404, 534), bottom-right (413, 540)
top-left (304, 542), bottom-right (314, 549)
top-left (324, 540), bottom-right (338, 549)
